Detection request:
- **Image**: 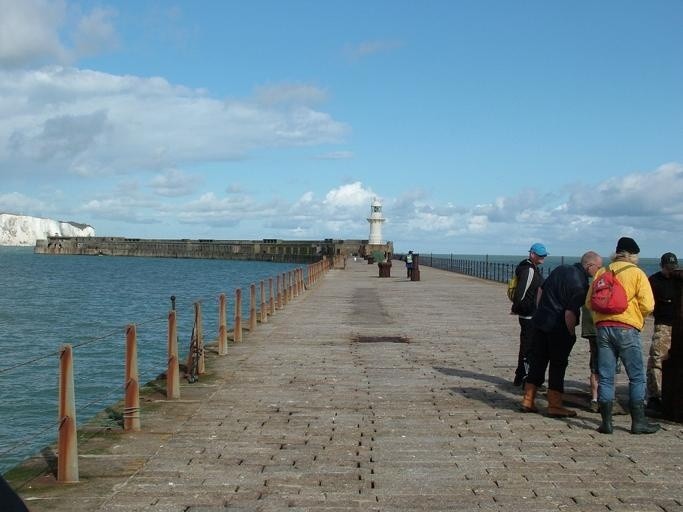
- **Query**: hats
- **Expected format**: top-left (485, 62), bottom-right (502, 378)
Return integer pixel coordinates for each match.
top-left (615, 237), bottom-right (639, 255)
top-left (660, 252), bottom-right (677, 268)
top-left (527, 242), bottom-right (547, 257)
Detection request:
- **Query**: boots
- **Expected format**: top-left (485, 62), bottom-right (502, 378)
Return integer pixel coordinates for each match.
top-left (629, 398), bottom-right (659, 434)
top-left (546, 389), bottom-right (576, 418)
top-left (520, 383), bottom-right (537, 413)
top-left (597, 401), bottom-right (612, 434)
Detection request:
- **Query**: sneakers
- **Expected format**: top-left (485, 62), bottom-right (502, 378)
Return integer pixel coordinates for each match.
top-left (513, 374), bottom-right (522, 386)
top-left (589, 400), bottom-right (600, 412)
top-left (611, 400), bottom-right (628, 416)
top-left (522, 377), bottom-right (546, 392)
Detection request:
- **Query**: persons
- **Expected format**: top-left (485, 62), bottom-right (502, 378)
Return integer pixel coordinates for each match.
top-left (583, 236), bottom-right (663, 434)
top-left (403, 250), bottom-right (414, 280)
top-left (579, 304), bottom-right (609, 414)
top-left (518, 250), bottom-right (603, 420)
top-left (508, 242), bottom-right (551, 388)
top-left (647, 250), bottom-right (682, 418)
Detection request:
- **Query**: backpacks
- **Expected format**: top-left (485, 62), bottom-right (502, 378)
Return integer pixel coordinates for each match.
top-left (505, 275), bottom-right (517, 301)
top-left (590, 264), bottom-right (642, 315)
